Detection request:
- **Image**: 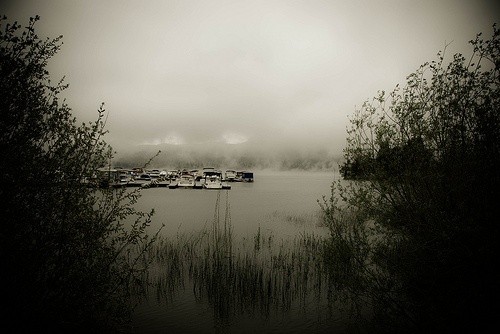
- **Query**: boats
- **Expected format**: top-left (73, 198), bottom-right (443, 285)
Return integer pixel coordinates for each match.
top-left (90, 157), bottom-right (258, 194)
